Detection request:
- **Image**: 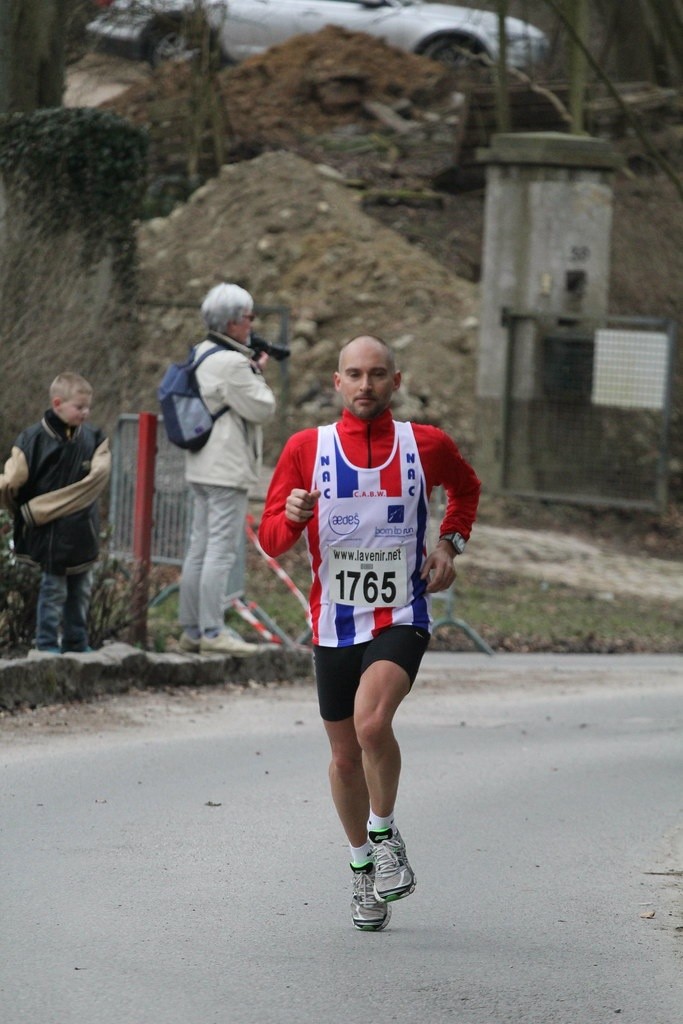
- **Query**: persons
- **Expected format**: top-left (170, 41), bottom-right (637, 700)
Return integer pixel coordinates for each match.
top-left (177, 280), bottom-right (277, 654)
top-left (0, 371), bottom-right (112, 655)
top-left (257, 336), bottom-right (481, 932)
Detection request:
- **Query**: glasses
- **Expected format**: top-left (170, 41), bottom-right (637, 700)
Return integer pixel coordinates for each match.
top-left (242, 315), bottom-right (255, 321)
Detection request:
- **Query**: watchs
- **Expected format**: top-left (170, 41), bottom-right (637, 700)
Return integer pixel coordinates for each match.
top-left (440, 531), bottom-right (467, 554)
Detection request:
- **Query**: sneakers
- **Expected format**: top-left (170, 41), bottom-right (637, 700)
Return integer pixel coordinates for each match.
top-left (200, 629), bottom-right (258, 657)
top-left (350, 861), bottom-right (392, 931)
top-left (369, 827), bottom-right (417, 903)
top-left (176, 631), bottom-right (201, 654)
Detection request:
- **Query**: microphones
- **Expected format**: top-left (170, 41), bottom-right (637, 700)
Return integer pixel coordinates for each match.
top-left (247, 333), bottom-right (290, 362)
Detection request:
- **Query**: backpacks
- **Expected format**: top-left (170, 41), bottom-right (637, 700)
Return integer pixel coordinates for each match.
top-left (157, 344), bottom-right (230, 448)
